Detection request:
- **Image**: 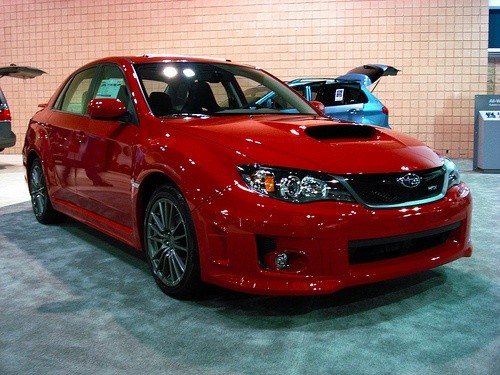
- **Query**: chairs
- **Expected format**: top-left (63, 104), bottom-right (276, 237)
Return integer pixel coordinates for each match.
top-left (150, 92), bottom-right (176, 116)
top-left (179, 81), bottom-right (221, 113)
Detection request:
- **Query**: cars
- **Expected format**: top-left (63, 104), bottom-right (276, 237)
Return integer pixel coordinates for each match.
top-left (0, 63), bottom-right (48, 153)
top-left (21, 52), bottom-right (474, 300)
top-left (218, 62), bottom-right (402, 132)
